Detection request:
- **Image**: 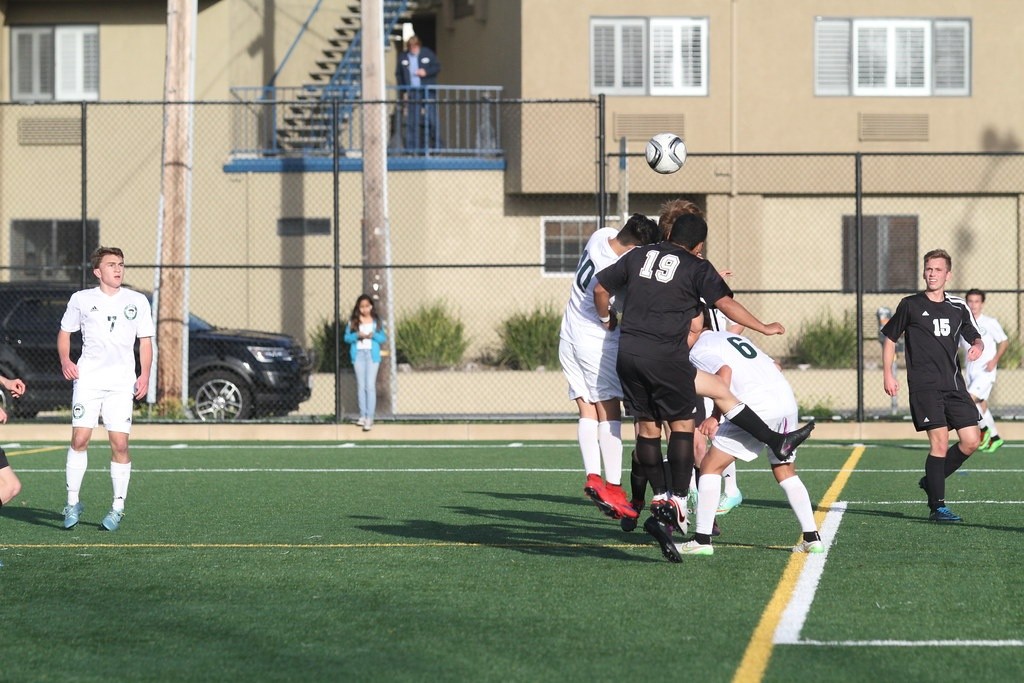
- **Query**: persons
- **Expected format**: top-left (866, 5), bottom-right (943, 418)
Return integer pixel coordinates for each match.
top-left (57, 246), bottom-right (153, 531)
top-left (558, 199), bottom-right (824, 564)
top-left (344, 295), bottom-right (387, 431)
top-left (395, 36), bottom-right (443, 157)
top-left (880, 250), bottom-right (984, 522)
top-left (0, 375), bottom-right (24, 511)
top-left (958, 289), bottom-right (1008, 454)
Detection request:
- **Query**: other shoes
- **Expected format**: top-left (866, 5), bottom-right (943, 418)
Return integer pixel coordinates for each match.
top-left (358, 418), bottom-right (365, 425)
top-left (364, 421), bottom-right (372, 431)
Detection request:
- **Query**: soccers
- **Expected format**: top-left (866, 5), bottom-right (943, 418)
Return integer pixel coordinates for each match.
top-left (645, 132), bottom-right (688, 175)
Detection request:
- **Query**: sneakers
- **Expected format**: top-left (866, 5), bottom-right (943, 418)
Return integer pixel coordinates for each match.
top-left (100, 510), bottom-right (124, 532)
top-left (929, 506), bottom-right (963, 521)
top-left (62, 501), bottom-right (84, 529)
top-left (774, 420), bottom-right (815, 462)
top-left (919, 476), bottom-right (927, 494)
top-left (688, 491), bottom-right (698, 514)
top-left (716, 489), bottom-right (743, 515)
top-left (621, 499), bottom-right (646, 532)
top-left (607, 488), bottom-right (639, 520)
top-left (674, 539), bottom-right (714, 555)
top-left (785, 540), bottom-right (824, 553)
top-left (977, 427), bottom-right (991, 450)
top-left (712, 521), bottom-right (720, 536)
top-left (643, 516), bottom-right (683, 563)
top-left (985, 438), bottom-right (1004, 453)
top-left (584, 479), bottom-right (624, 519)
top-left (651, 491), bottom-right (688, 535)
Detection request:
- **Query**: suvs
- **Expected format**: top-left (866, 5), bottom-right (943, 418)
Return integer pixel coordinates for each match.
top-left (0, 280), bottom-right (314, 424)
